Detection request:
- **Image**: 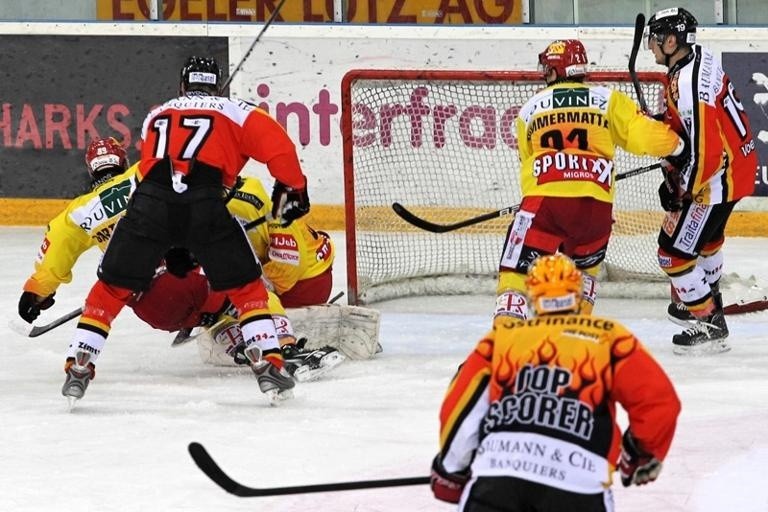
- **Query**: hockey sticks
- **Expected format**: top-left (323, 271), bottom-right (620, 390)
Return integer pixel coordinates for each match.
top-left (629, 12), bottom-right (650, 113)
top-left (393, 160), bottom-right (669, 233)
top-left (188, 442), bottom-right (624, 496)
top-left (3, 201), bottom-right (301, 338)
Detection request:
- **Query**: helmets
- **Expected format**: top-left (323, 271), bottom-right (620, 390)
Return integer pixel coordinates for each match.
top-left (525, 252), bottom-right (582, 315)
top-left (179, 55), bottom-right (222, 96)
top-left (83, 137), bottom-right (129, 185)
top-left (642, 8), bottom-right (697, 51)
top-left (536, 38), bottom-right (588, 83)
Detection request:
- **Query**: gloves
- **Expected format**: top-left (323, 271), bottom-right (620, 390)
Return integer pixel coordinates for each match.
top-left (271, 177), bottom-right (313, 227)
top-left (18, 291), bottom-right (57, 325)
top-left (428, 455), bottom-right (474, 503)
top-left (658, 176), bottom-right (681, 213)
top-left (165, 248), bottom-right (197, 278)
top-left (666, 132), bottom-right (691, 172)
top-left (619, 428), bottom-right (661, 488)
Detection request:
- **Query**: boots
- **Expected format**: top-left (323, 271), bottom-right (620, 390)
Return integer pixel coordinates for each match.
top-left (667, 277), bottom-right (722, 320)
top-left (62, 352), bottom-right (96, 399)
top-left (672, 293), bottom-right (726, 345)
top-left (230, 339), bottom-right (338, 391)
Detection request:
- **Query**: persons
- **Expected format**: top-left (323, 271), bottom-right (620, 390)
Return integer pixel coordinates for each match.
top-left (429, 254), bottom-right (681, 512)
top-left (493, 39), bottom-right (687, 326)
top-left (646, 6), bottom-right (758, 356)
top-left (17, 137), bottom-right (344, 382)
top-left (61, 55), bottom-right (311, 408)
top-left (224, 175), bottom-right (335, 306)
